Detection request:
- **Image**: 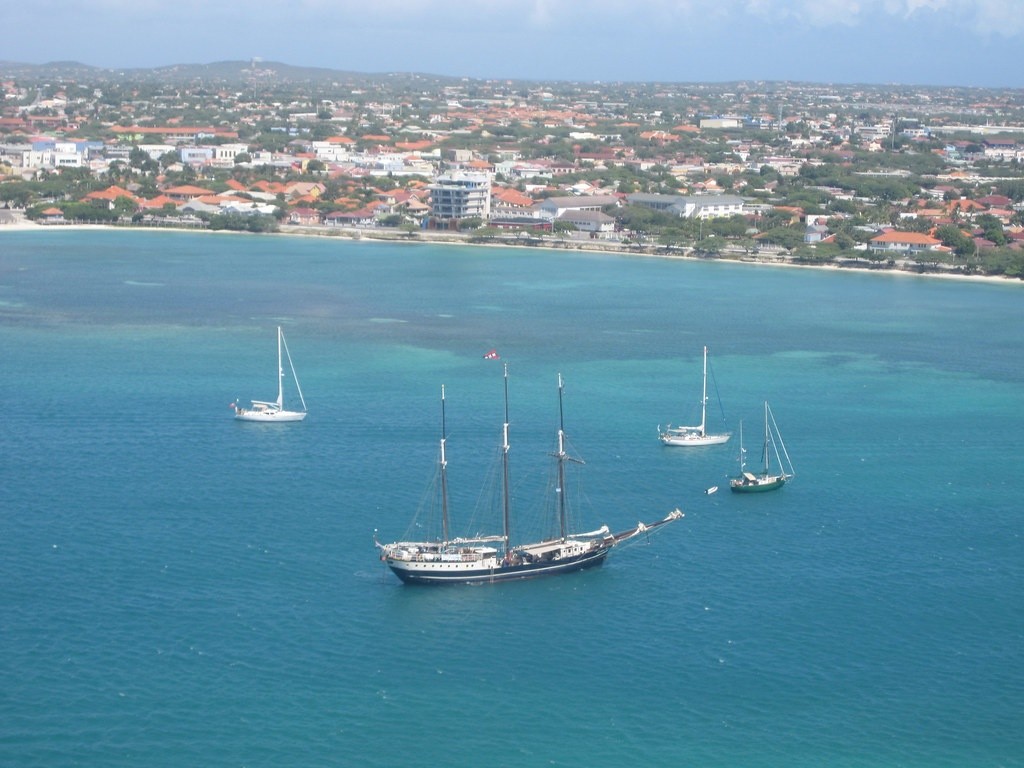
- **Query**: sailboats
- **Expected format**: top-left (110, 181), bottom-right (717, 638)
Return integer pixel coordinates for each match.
top-left (728, 400), bottom-right (797, 494)
top-left (371, 360), bottom-right (687, 584)
top-left (657, 344), bottom-right (731, 446)
top-left (230, 324), bottom-right (310, 422)
